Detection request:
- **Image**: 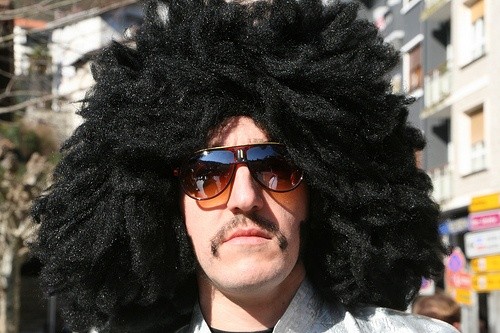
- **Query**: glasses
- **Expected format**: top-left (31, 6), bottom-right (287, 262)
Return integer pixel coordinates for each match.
top-left (173, 141), bottom-right (304, 200)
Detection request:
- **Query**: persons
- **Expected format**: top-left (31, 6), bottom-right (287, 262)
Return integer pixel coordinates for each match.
top-left (411, 293), bottom-right (461, 333)
top-left (29, 0), bottom-right (460, 333)
